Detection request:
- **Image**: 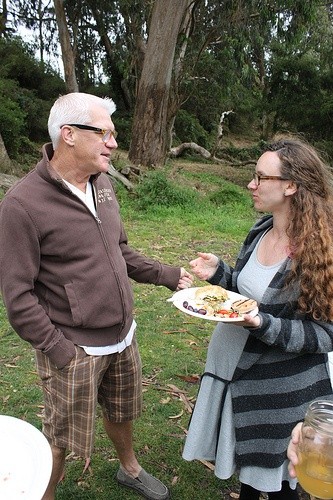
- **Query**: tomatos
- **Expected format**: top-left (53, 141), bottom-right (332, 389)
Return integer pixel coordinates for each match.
top-left (218, 310), bottom-right (239, 318)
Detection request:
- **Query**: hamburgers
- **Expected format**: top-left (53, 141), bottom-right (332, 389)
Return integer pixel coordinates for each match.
top-left (195, 286), bottom-right (228, 302)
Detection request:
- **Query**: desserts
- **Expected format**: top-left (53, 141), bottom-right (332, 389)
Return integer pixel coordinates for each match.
top-left (231, 298), bottom-right (258, 314)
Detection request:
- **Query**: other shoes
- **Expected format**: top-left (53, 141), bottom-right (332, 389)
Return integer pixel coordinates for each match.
top-left (116, 466), bottom-right (169, 500)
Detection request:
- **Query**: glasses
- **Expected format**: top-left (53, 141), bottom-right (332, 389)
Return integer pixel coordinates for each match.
top-left (252, 172), bottom-right (289, 185)
top-left (60, 124), bottom-right (119, 144)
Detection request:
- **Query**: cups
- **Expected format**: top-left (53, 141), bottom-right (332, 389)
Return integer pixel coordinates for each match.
top-left (293, 400), bottom-right (333, 500)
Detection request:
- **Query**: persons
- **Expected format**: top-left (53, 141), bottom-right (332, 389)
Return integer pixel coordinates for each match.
top-left (0, 91), bottom-right (194, 499)
top-left (285, 421), bottom-right (332, 480)
top-left (181, 131), bottom-right (332, 499)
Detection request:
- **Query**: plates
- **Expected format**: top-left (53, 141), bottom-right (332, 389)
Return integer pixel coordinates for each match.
top-left (0, 415), bottom-right (53, 499)
top-left (231, 299), bottom-right (257, 313)
top-left (172, 287), bottom-right (259, 322)
